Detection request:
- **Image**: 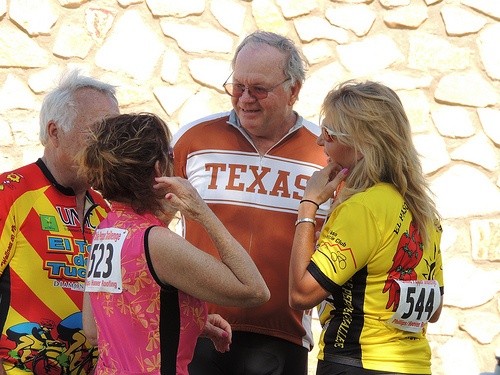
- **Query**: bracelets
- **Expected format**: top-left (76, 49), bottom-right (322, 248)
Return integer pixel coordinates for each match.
top-left (293, 217), bottom-right (317, 227)
top-left (299, 200), bottom-right (319, 208)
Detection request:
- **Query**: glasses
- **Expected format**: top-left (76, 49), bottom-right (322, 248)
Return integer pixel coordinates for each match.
top-left (321, 124), bottom-right (357, 142)
top-left (224, 70), bottom-right (292, 99)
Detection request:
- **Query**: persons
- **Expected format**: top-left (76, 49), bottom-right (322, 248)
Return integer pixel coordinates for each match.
top-left (169, 32), bottom-right (336, 375)
top-left (73, 112), bottom-right (270, 375)
top-left (0, 68), bottom-right (120, 375)
top-left (290, 79), bottom-right (445, 375)
